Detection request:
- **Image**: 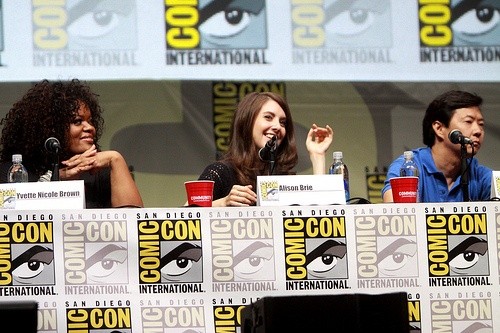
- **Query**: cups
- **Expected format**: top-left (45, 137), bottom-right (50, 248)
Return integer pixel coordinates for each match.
top-left (184, 180), bottom-right (215, 207)
top-left (389, 176), bottom-right (419, 203)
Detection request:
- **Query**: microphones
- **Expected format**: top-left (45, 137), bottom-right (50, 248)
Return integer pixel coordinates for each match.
top-left (448, 130), bottom-right (473, 144)
top-left (259, 136), bottom-right (280, 163)
top-left (44, 137), bottom-right (60, 153)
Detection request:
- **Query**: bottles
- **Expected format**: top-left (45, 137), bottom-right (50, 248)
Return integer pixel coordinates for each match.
top-left (329, 151), bottom-right (350, 205)
top-left (7, 154), bottom-right (29, 183)
top-left (400, 151), bottom-right (420, 177)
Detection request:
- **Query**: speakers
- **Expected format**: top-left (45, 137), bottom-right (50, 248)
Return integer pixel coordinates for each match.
top-left (240, 292), bottom-right (409, 333)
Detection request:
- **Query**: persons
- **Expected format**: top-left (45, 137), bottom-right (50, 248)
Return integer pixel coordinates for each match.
top-left (0, 80), bottom-right (143, 208)
top-left (184, 92), bottom-right (333, 208)
top-left (381, 90), bottom-right (492, 203)
